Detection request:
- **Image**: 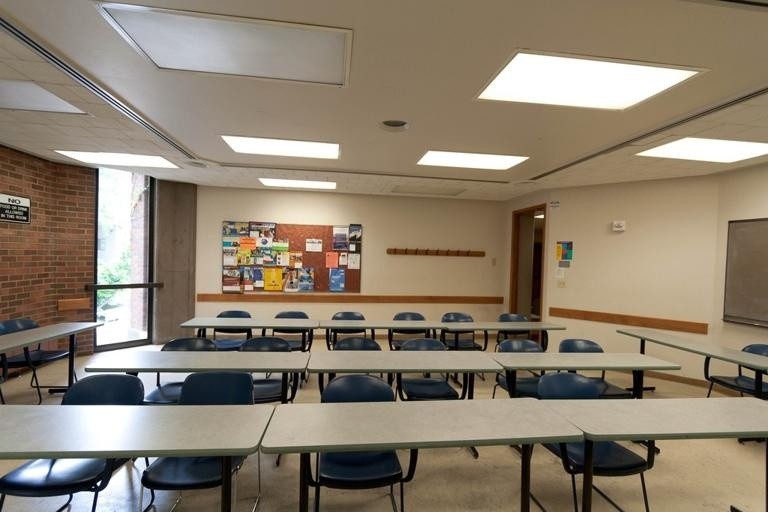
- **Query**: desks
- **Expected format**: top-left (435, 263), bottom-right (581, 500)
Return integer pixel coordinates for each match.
top-left (2, 320), bottom-right (103, 405)
top-left (85, 345), bottom-right (683, 454)
top-left (0, 396), bottom-right (767, 512)
top-left (179, 318), bottom-right (567, 377)
top-left (615, 327), bottom-right (766, 445)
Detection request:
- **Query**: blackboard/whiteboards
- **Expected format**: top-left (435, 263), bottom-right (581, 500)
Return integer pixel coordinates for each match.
top-left (722, 218), bottom-right (767, 327)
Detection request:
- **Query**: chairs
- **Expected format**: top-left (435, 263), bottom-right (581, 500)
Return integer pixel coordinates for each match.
top-left (213, 311), bottom-right (531, 378)
top-left (310, 374), bottom-right (421, 512)
top-left (2, 373), bottom-right (155, 512)
top-left (141, 373), bottom-right (262, 512)
top-left (528, 371), bottom-right (653, 511)
top-left (143, 337), bottom-right (630, 459)
top-left (1, 317), bottom-right (79, 406)
top-left (702, 344), bottom-right (766, 444)
